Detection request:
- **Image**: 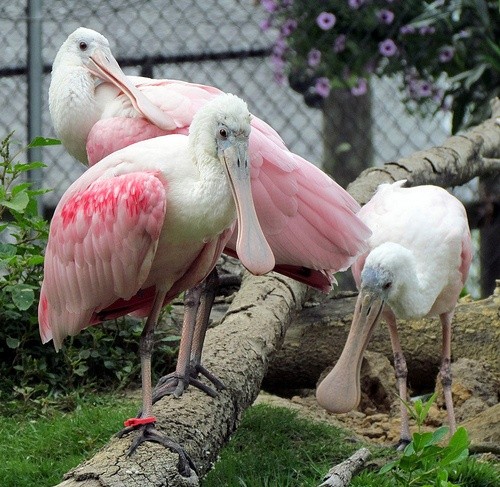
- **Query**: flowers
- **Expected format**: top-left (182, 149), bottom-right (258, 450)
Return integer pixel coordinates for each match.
top-left (259, 1), bottom-right (464, 111)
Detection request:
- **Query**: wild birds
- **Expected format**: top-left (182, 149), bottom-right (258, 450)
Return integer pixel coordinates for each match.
top-left (49, 27), bottom-right (374, 401)
top-left (315, 180), bottom-right (473, 449)
top-left (37, 93), bottom-right (276, 476)
